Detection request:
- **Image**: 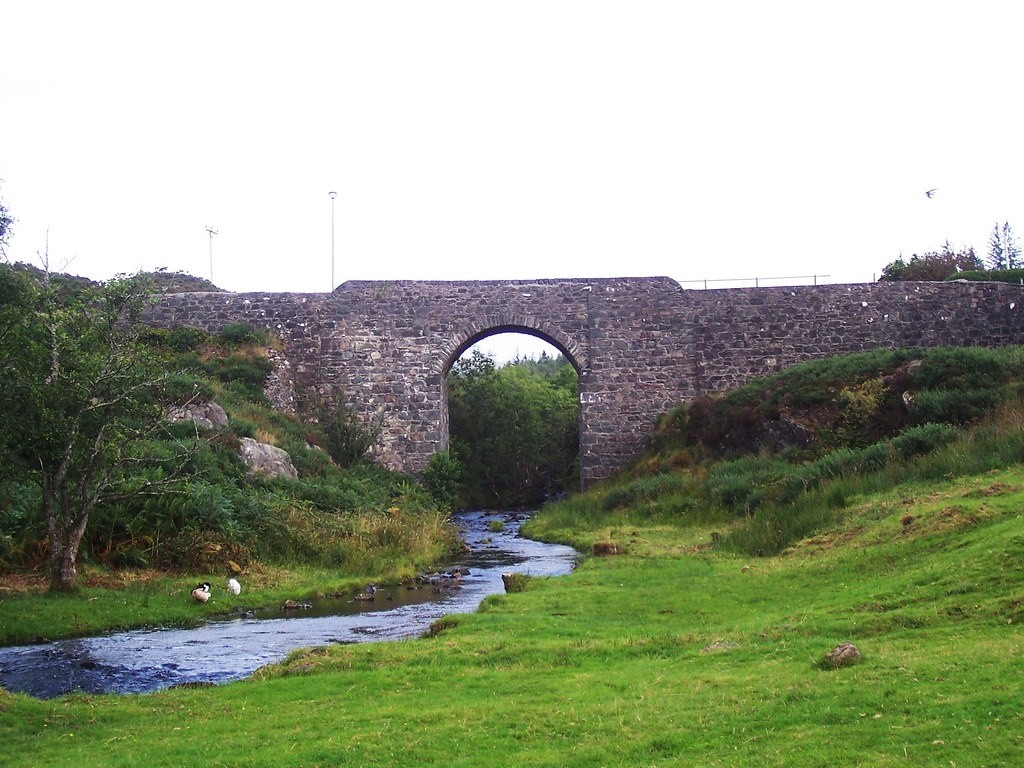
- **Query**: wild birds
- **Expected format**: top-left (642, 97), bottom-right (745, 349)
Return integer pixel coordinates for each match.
top-left (386, 507), bottom-right (400, 517)
top-left (306, 430), bottom-right (317, 448)
top-left (365, 583), bottom-right (377, 598)
top-left (191, 542), bottom-right (241, 603)
